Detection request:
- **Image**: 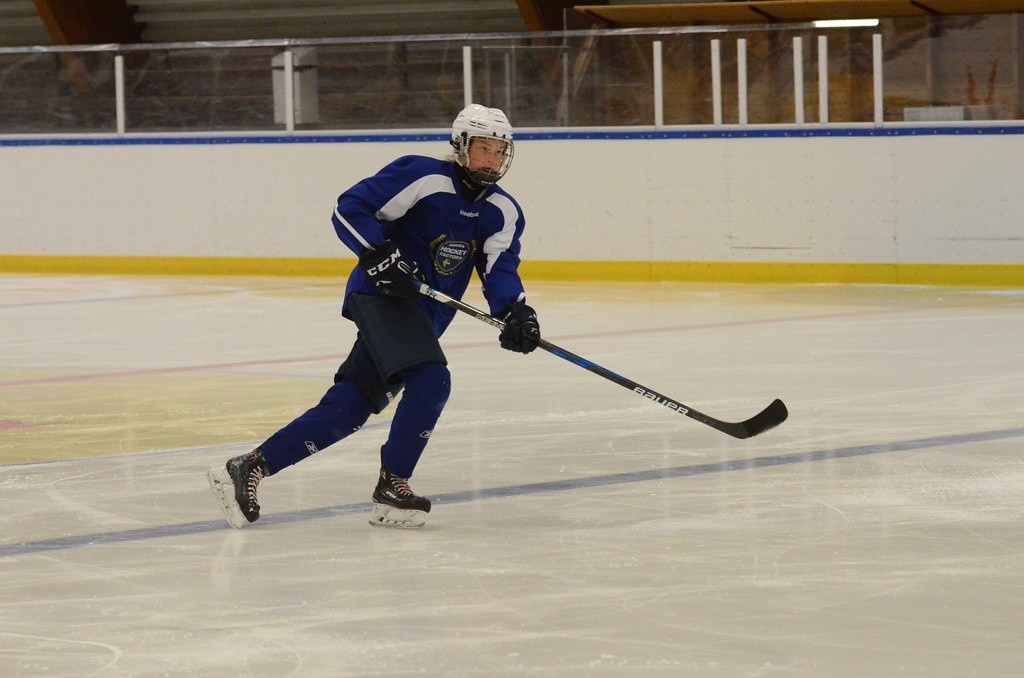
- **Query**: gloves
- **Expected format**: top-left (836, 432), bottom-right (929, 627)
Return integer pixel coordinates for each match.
top-left (497, 302), bottom-right (541, 355)
top-left (359, 240), bottom-right (428, 300)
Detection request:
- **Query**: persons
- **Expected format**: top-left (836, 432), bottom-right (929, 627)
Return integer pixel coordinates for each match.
top-left (208, 103), bottom-right (540, 527)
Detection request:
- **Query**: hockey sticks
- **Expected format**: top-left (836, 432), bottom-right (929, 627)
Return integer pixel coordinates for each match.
top-left (415, 281), bottom-right (789, 441)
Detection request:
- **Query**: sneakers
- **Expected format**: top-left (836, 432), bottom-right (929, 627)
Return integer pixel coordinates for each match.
top-left (369, 446), bottom-right (432, 529)
top-left (206, 446), bottom-right (269, 530)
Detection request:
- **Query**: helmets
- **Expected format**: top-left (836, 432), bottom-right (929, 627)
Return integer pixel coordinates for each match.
top-left (450, 103), bottom-right (513, 167)
